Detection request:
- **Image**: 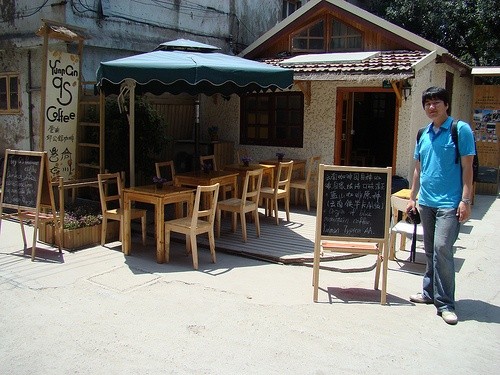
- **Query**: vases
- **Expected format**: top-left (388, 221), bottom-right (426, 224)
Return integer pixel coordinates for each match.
top-left (244, 161), bottom-right (249, 167)
top-left (278, 156), bottom-right (283, 162)
top-left (204, 169), bottom-right (209, 176)
top-left (157, 183), bottom-right (163, 189)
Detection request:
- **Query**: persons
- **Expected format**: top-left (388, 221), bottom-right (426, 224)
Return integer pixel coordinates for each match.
top-left (405, 88), bottom-right (476, 324)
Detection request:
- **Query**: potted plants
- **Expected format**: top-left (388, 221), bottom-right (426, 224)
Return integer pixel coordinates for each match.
top-left (208, 126), bottom-right (219, 144)
top-left (38, 206), bottom-right (119, 251)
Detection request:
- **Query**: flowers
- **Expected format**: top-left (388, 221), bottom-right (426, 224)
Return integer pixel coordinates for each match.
top-left (205, 160), bottom-right (213, 170)
top-left (240, 156), bottom-right (252, 162)
top-left (276, 151), bottom-right (285, 157)
top-left (154, 176), bottom-right (169, 183)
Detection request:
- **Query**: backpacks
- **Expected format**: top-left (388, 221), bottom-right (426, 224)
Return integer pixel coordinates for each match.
top-left (415, 119), bottom-right (478, 184)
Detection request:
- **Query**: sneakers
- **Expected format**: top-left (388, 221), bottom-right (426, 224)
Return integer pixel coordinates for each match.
top-left (410, 292), bottom-right (427, 303)
top-left (442, 310), bottom-right (458, 324)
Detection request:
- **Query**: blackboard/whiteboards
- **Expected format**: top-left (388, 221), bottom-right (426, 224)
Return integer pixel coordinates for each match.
top-left (315, 163), bottom-right (392, 244)
top-left (0, 149), bottom-right (56, 213)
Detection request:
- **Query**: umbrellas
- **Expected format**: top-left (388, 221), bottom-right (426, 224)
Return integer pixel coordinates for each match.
top-left (94, 39), bottom-right (293, 173)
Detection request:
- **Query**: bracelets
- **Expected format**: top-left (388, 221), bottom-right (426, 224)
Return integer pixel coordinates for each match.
top-left (462, 199), bottom-right (472, 204)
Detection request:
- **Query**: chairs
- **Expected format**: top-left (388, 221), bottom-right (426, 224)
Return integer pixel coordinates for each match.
top-left (390, 176), bottom-right (409, 221)
top-left (98, 155), bottom-right (322, 270)
top-left (388, 196), bottom-right (425, 276)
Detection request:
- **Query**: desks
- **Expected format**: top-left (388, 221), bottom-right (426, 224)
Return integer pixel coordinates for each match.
top-left (120, 184), bottom-right (196, 264)
top-left (174, 170), bottom-right (240, 235)
top-left (389, 189), bottom-right (419, 261)
top-left (224, 164), bottom-right (276, 223)
top-left (260, 159), bottom-right (307, 209)
